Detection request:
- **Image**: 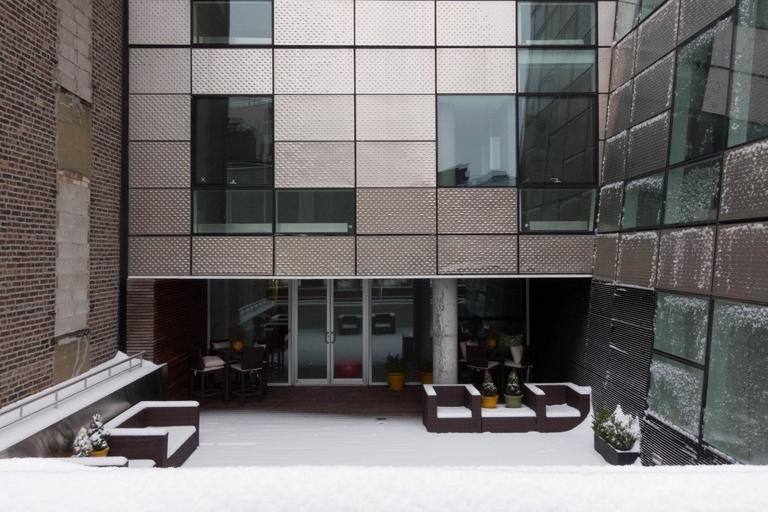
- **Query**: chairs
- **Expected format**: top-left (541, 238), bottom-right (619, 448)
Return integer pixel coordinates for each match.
top-left (458, 335), bottom-right (532, 379)
top-left (103, 401), bottom-right (200, 470)
top-left (192, 339), bottom-right (270, 405)
top-left (267, 332), bottom-right (288, 375)
top-left (423, 383), bottom-right (480, 433)
top-left (525, 371), bottom-right (589, 431)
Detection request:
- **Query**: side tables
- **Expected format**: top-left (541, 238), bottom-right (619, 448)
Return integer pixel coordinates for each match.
top-left (481, 403), bottom-right (536, 432)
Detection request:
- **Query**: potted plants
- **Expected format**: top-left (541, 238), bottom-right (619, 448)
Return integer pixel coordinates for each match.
top-left (87, 412), bottom-right (110, 457)
top-left (416, 353), bottom-right (432, 384)
top-left (385, 353), bottom-right (405, 391)
top-left (482, 369), bottom-right (502, 409)
top-left (506, 372), bottom-right (522, 407)
top-left (591, 405), bottom-right (642, 465)
